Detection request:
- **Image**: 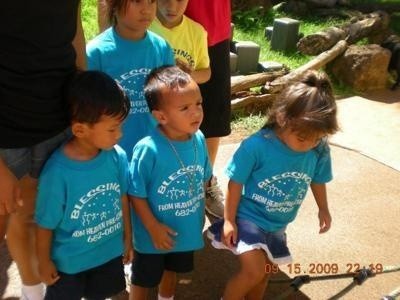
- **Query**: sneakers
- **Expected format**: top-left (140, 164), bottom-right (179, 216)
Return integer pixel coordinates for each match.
top-left (205, 175), bottom-right (226, 219)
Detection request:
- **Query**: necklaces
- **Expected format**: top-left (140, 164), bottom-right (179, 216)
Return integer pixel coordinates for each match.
top-left (159, 128), bottom-right (198, 191)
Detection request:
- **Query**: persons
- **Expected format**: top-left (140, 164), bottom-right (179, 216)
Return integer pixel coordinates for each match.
top-left (147, 0), bottom-right (212, 86)
top-left (0, 1), bottom-right (87, 300)
top-left (127, 63), bottom-right (228, 300)
top-left (206, 69), bottom-right (340, 300)
top-left (32, 69), bottom-right (134, 300)
top-left (85, 0), bottom-right (176, 164)
top-left (97, 0), bottom-right (233, 221)
